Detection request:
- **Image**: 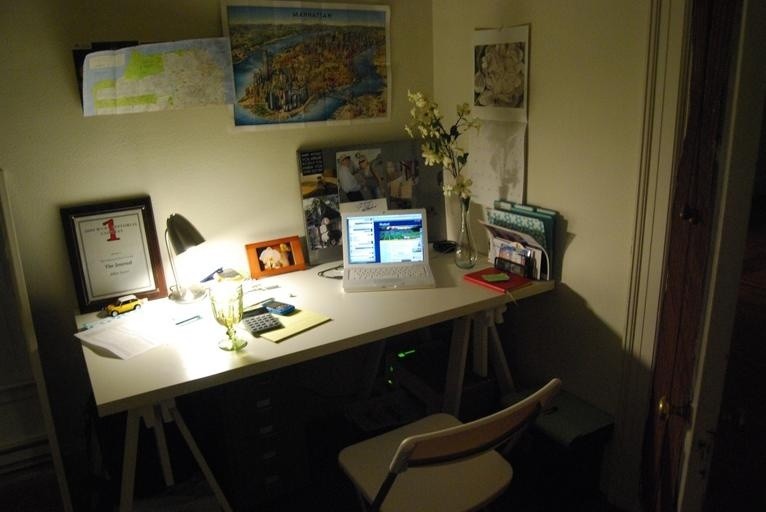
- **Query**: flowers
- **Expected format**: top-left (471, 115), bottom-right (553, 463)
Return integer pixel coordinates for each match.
top-left (405, 93), bottom-right (480, 268)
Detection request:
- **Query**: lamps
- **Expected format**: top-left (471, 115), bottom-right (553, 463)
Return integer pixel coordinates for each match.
top-left (161, 208), bottom-right (207, 302)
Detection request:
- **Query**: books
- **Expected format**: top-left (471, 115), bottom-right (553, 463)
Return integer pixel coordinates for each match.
top-left (478, 199), bottom-right (557, 284)
top-left (463, 267), bottom-right (532, 294)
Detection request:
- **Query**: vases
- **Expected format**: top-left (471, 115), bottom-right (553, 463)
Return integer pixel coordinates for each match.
top-left (451, 187), bottom-right (473, 268)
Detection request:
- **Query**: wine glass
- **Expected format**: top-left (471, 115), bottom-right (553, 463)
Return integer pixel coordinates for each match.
top-left (206, 279), bottom-right (248, 351)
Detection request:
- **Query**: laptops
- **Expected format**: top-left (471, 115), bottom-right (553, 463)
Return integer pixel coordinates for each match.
top-left (341, 209), bottom-right (436, 292)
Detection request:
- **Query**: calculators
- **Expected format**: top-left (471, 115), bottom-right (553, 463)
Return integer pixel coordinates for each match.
top-left (244, 307), bottom-right (280, 334)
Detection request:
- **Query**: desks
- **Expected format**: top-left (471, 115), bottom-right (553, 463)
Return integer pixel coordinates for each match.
top-left (73, 244), bottom-right (555, 511)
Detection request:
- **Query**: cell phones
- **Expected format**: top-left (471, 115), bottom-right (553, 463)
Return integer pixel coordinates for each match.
top-left (262, 301), bottom-right (294, 314)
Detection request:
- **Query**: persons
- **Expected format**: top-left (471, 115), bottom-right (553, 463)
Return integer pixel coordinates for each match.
top-left (356, 156), bottom-right (385, 199)
top-left (337, 153), bottom-right (365, 203)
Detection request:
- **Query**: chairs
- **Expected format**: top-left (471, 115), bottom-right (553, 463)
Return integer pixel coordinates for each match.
top-left (336, 376), bottom-right (559, 511)
